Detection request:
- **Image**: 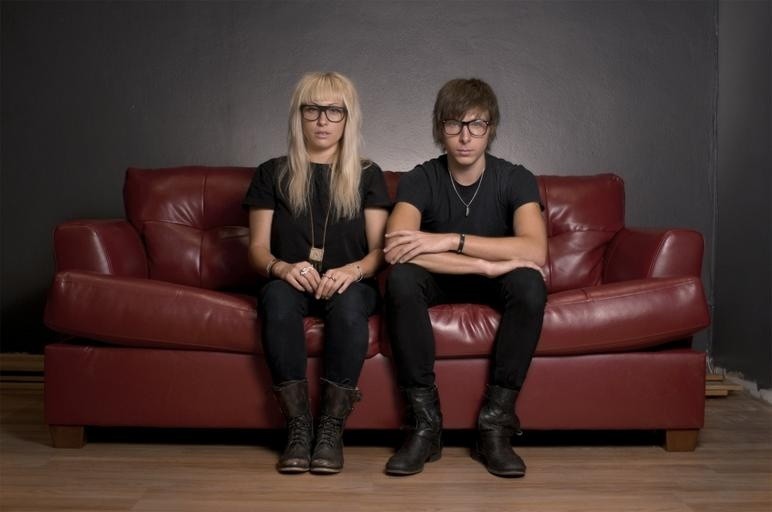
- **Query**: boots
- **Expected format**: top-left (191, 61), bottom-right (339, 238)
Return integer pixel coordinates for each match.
top-left (270, 378), bottom-right (309, 473)
top-left (468, 383), bottom-right (528, 477)
top-left (310, 378), bottom-right (363, 474)
top-left (384, 386), bottom-right (444, 477)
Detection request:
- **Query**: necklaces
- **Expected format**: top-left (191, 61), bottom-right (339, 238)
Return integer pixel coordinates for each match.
top-left (308, 195), bottom-right (332, 272)
top-left (446, 166), bottom-right (487, 218)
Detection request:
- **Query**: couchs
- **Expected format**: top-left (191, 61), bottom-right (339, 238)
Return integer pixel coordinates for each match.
top-left (43, 168), bottom-right (712, 452)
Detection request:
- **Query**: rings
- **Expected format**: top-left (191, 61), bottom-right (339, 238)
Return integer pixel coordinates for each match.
top-left (300, 267), bottom-right (309, 275)
top-left (322, 273), bottom-right (329, 278)
top-left (329, 277), bottom-right (336, 282)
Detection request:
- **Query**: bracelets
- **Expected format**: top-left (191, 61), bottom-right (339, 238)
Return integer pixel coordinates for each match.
top-left (456, 232), bottom-right (465, 253)
top-left (350, 262), bottom-right (365, 283)
top-left (265, 258), bottom-right (281, 277)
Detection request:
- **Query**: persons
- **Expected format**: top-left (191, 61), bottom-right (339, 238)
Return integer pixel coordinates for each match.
top-left (238, 70), bottom-right (393, 477)
top-left (380, 74), bottom-right (551, 479)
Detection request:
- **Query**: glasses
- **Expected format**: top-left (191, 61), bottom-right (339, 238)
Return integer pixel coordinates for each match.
top-left (439, 117), bottom-right (496, 137)
top-left (299, 105), bottom-right (347, 122)
